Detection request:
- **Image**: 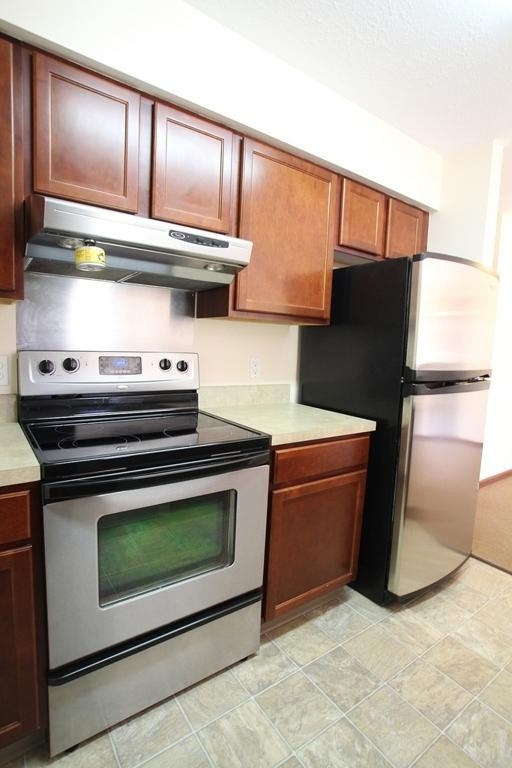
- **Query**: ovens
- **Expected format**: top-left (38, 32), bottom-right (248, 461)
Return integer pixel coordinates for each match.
top-left (30, 446), bottom-right (276, 760)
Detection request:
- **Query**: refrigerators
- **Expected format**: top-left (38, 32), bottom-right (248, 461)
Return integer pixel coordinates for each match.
top-left (298, 254), bottom-right (499, 608)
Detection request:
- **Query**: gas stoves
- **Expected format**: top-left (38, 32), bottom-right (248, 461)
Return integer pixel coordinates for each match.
top-left (15, 348), bottom-right (273, 483)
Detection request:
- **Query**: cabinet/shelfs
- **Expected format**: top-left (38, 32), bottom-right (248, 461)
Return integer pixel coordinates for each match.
top-left (194, 100), bottom-right (336, 327)
top-left (0, 422), bottom-right (40, 768)
top-left (0, 19), bottom-right (26, 301)
top-left (207, 402), bottom-right (377, 637)
top-left (335, 165), bottom-right (430, 261)
top-left (23, 24), bottom-right (237, 235)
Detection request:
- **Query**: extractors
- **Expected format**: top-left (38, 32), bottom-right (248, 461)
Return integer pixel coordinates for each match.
top-left (22, 193), bottom-right (254, 293)
